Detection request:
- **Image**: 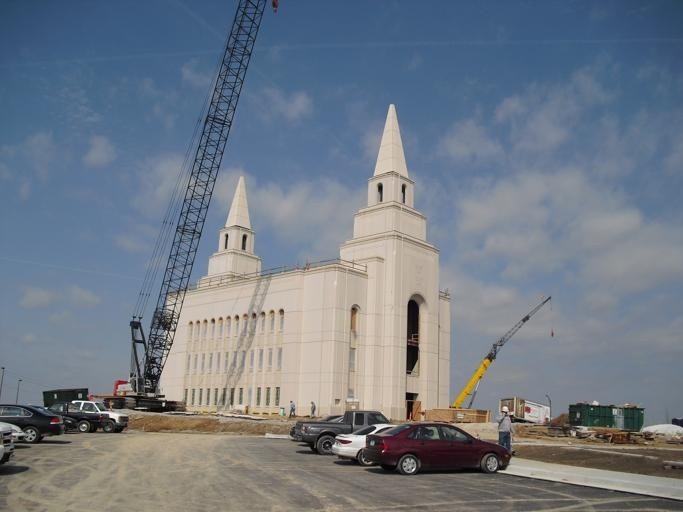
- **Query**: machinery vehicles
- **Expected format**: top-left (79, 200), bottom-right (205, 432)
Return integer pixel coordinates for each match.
top-left (92, 0), bottom-right (267, 412)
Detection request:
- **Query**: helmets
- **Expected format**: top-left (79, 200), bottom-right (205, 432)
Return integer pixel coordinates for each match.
top-left (501, 406), bottom-right (509, 412)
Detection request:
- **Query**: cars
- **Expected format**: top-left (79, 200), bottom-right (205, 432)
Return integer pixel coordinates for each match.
top-left (365, 422), bottom-right (511, 476)
top-left (0, 400), bottom-right (128, 465)
top-left (329, 422), bottom-right (460, 466)
top-left (290, 415), bottom-right (344, 440)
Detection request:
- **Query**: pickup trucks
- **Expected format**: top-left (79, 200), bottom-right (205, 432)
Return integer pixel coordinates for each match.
top-left (293, 410), bottom-right (393, 456)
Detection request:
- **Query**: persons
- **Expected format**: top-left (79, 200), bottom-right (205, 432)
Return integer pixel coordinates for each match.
top-left (288, 401), bottom-right (296, 418)
top-left (310, 402), bottom-right (316, 419)
top-left (496, 406), bottom-right (515, 454)
